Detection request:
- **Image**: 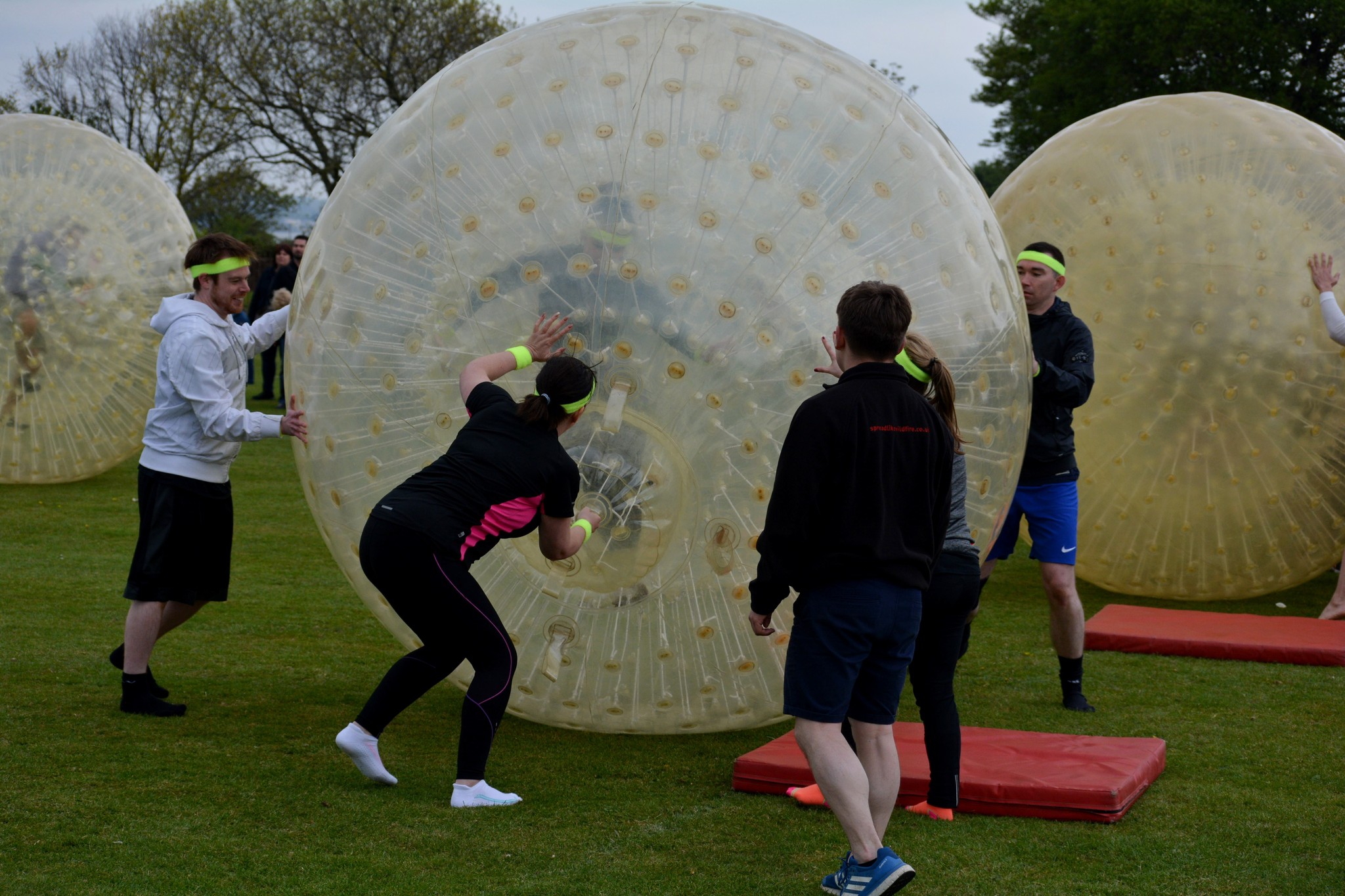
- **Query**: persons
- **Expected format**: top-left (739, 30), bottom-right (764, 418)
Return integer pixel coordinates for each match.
top-left (980, 243), bottom-right (1099, 714)
top-left (107, 231), bottom-right (309, 717)
top-left (247, 234), bottom-right (309, 410)
top-left (747, 282), bottom-right (954, 896)
top-left (333, 310), bottom-right (603, 807)
top-left (786, 334), bottom-right (981, 822)
top-left (450, 183), bottom-right (723, 548)
top-left (1308, 251), bottom-right (1345, 620)
top-left (0, 216), bottom-right (89, 430)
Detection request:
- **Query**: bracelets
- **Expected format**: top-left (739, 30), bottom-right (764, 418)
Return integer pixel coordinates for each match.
top-left (1032, 362), bottom-right (1040, 377)
top-left (569, 519), bottom-right (592, 544)
top-left (506, 344), bottom-right (533, 371)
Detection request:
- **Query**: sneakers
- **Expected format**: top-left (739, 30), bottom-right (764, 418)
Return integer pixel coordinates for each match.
top-left (820, 850), bottom-right (857, 896)
top-left (840, 846), bottom-right (916, 896)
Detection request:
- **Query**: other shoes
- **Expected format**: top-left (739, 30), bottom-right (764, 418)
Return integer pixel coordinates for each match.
top-left (1062, 694), bottom-right (1096, 713)
